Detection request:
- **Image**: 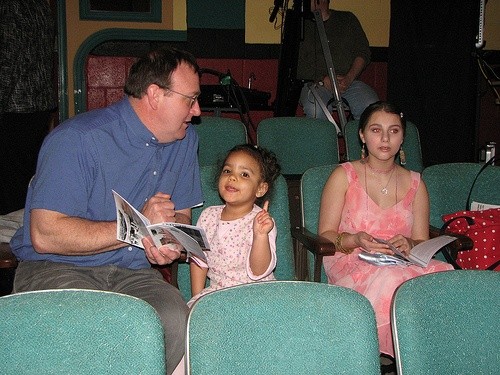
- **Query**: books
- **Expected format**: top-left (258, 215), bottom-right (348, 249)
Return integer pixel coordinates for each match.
top-left (112, 190), bottom-right (210, 263)
top-left (358, 235), bottom-right (457, 268)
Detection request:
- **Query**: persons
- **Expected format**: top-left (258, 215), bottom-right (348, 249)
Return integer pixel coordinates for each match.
top-left (299, 0), bottom-right (380, 123)
top-left (187, 144), bottom-right (280, 309)
top-left (318, 100), bottom-right (455, 357)
top-left (9, 47), bottom-right (201, 375)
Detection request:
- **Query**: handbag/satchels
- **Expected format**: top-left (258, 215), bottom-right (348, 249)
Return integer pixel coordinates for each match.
top-left (439, 155), bottom-right (500, 271)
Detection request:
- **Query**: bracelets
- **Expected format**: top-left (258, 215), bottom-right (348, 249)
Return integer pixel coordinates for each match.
top-left (335, 232), bottom-right (355, 255)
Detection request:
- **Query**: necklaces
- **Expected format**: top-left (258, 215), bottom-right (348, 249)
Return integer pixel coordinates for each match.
top-left (369, 165), bottom-right (395, 195)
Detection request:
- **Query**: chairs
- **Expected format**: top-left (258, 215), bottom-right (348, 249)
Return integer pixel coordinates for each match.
top-left (0, 116), bottom-right (500, 375)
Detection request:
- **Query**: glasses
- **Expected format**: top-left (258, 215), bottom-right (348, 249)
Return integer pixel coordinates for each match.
top-left (165, 88), bottom-right (199, 108)
